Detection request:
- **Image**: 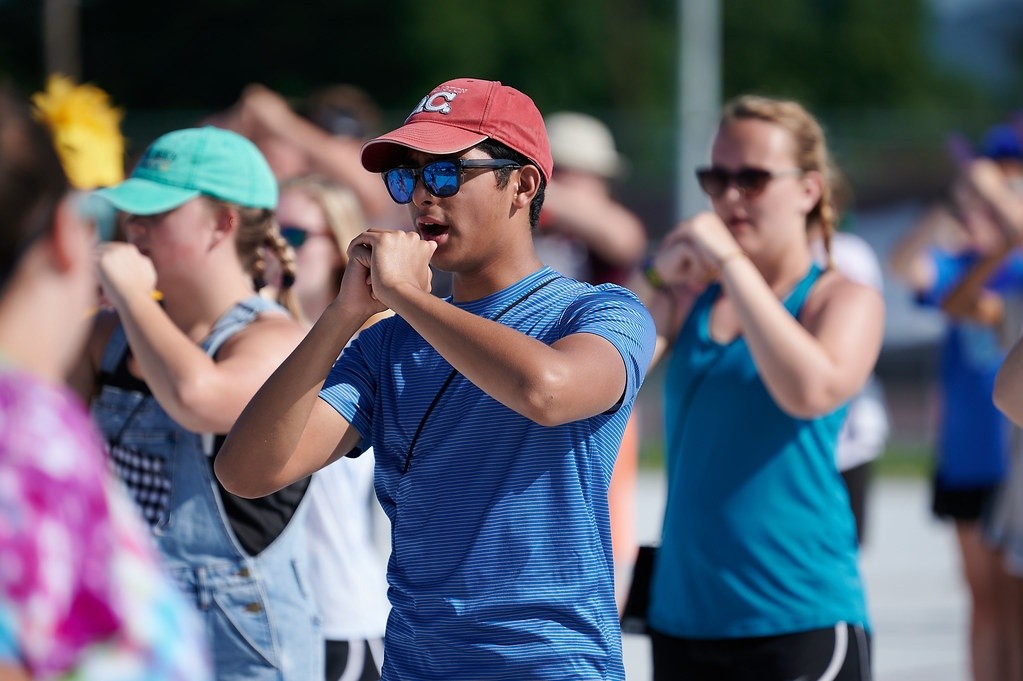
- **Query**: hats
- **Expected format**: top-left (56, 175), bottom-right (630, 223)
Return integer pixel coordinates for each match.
top-left (543, 110), bottom-right (619, 177)
top-left (88, 125), bottom-right (279, 216)
top-left (360, 77), bottom-right (554, 186)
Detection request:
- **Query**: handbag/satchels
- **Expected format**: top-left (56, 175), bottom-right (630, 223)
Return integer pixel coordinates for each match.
top-left (622, 547), bottom-right (657, 635)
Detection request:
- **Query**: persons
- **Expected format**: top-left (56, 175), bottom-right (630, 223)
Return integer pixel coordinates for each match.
top-left (0, 86), bottom-right (646, 681)
top-left (893, 123), bottom-right (1023, 681)
top-left (212, 78), bottom-right (655, 681)
top-left (618, 93), bottom-right (890, 681)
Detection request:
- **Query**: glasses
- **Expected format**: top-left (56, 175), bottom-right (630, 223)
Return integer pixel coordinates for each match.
top-left (276, 226), bottom-right (332, 250)
top-left (695, 167), bottom-right (806, 198)
top-left (381, 158), bottom-right (528, 205)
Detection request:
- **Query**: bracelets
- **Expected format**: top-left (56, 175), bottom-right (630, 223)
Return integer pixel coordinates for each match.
top-left (638, 248), bottom-right (665, 288)
